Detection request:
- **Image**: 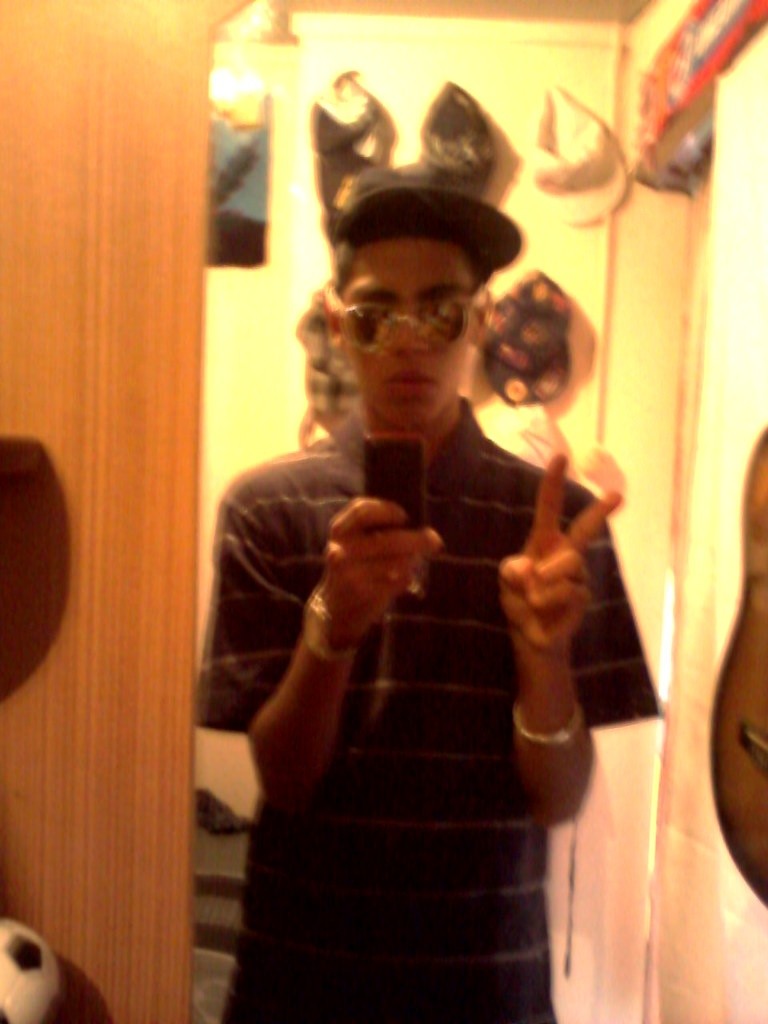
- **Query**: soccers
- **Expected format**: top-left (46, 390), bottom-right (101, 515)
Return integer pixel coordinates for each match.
top-left (0, 917), bottom-right (64, 1024)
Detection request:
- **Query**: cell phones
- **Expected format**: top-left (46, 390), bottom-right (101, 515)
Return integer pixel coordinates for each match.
top-left (360, 436), bottom-right (428, 536)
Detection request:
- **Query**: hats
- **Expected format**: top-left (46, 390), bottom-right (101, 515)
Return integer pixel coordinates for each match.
top-left (418, 83), bottom-right (499, 191)
top-left (481, 270), bottom-right (574, 405)
top-left (332, 165), bottom-right (520, 274)
top-left (311, 71), bottom-right (395, 214)
top-left (535, 86), bottom-right (631, 227)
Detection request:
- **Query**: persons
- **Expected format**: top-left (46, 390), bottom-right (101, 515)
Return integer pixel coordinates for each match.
top-left (194, 181), bottom-right (659, 1023)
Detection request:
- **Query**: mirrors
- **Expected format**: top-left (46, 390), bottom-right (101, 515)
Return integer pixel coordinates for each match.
top-left (190, 3), bottom-right (725, 1021)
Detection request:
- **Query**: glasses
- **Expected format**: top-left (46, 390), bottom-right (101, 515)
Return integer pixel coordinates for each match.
top-left (340, 298), bottom-right (482, 354)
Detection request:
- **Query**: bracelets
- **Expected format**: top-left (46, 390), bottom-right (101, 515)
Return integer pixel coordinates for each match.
top-left (513, 696), bottom-right (585, 744)
top-left (305, 595), bottom-right (355, 661)
